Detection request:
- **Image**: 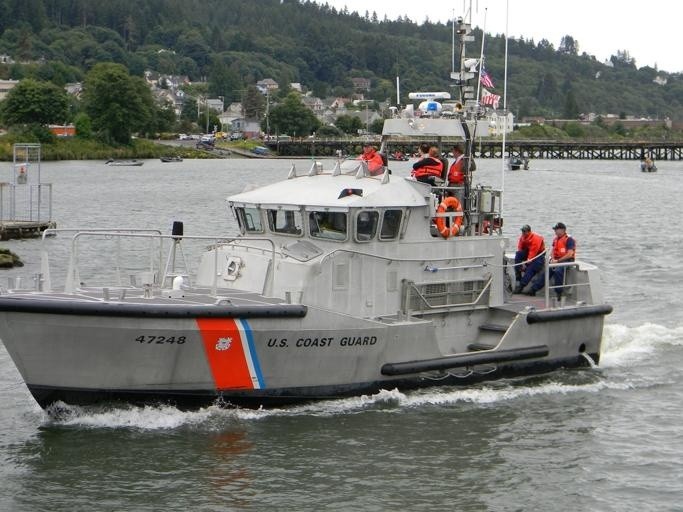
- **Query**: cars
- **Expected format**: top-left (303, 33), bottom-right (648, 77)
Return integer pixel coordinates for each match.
top-left (178, 132), bottom-right (245, 151)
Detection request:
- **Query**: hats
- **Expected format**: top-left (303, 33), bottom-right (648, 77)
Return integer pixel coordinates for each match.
top-left (520, 225), bottom-right (531, 232)
top-left (553, 222), bottom-right (566, 229)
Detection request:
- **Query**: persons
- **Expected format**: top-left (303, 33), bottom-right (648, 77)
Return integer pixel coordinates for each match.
top-left (407, 143), bottom-right (477, 223)
top-left (644, 158), bottom-right (652, 172)
top-left (513, 224), bottom-right (545, 295)
top-left (524, 221), bottom-right (576, 301)
top-left (354, 141), bottom-right (385, 175)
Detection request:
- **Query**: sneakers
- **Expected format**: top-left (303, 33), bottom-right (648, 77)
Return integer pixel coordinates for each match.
top-left (521, 287), bottom-right (536, 297)
top-left (507, 284), bottom-right (523, 294)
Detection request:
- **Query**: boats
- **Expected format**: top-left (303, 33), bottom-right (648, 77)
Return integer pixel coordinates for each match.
top-left (641, 164), bottom-right (658, 172)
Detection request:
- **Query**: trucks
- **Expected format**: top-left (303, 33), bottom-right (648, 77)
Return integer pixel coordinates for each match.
top-left (250, 146), bottom-right (269, 156)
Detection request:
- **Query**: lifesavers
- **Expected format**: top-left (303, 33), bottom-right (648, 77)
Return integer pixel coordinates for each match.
top-left (437, 197), bottom-right (463, 237)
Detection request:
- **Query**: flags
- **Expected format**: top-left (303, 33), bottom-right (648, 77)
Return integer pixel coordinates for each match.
top-left (479, 70), bottom-right (496, 90)
top-left (479, 88), bottom-right (501, 110)
